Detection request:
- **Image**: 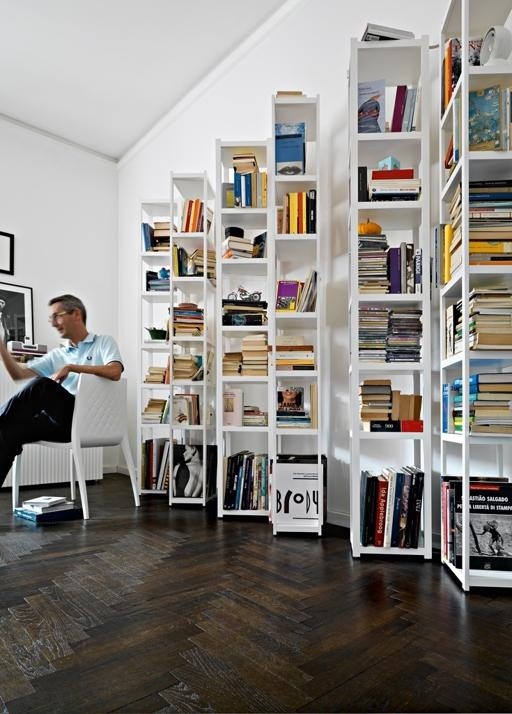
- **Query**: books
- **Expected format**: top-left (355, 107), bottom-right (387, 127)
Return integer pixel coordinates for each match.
top-left (357, 79), bottom-right (420, 133)
top-left (141, 395), bottom-right (170, 424)
top-left (357, 235), bottom-right (422, 294)
top-left (359, 379), bottom-right (423, 432)
top-left (222, 450), bottom-right (268, 510)
top-left (173, 393), bottom-right (199, 425)
top-left (222, 390), bottom-right (268, 426)
top-left (222, 153), bottom-right (266, 208)
top-left (222, 333), bottom-right (268, 376)
top-left (181, 198), bottom-right (213, 232)
top-left (142, 222), bottom-right (177, 252)
top-left (15, 495), bottom-right (83, 523)
top-left (274, 271), bottom-right (317, 312)
top-left (172, 244), bottom-right (216, 278)
top-left (173, 353), bottom-right (203, 381)
top-left (276, 335), bottom-right (315, 371)
top-left (360, 464), bottom-right (424, 549)
top-left (141, 438), bottom-right (170, 490)
top-left (275, 384), bottom-right (318, 429)
top-left (357, 166), bottom-right (422, 202)
top-left (145, 268), bottom-right (170, 292)
top-left (445, 84), bottom-right (510, 169)
top-left (440, 476), bottom-right (512, 571)
top-left (445, 285), bottom-right (512, 359)
top-left (222, 299), bottom-right (268, 326)
top-left (448, 180), bottom-right (512, 286)
top-left (360, 22), bottom-right (416, 42)
top-left (275, 122), bottom-right (305, 176)
top-left (276, 190), bottom-right (316, 234)
top-left (275, 89), bottom-right (303, 98)
top-left (221, 232), bottom-right (266, 258)
top-left (144, 355), bottom-right (170, 384)
top-left (173, 302), bottom-right (204, 336)
top-left (359, 305), bottom-right (423, 363)
top-left (442, 372), bottom-right (512, 433)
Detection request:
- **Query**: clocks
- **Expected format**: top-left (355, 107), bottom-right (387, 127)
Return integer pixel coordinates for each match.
top-left (479, 24), bottom-right (511, 67)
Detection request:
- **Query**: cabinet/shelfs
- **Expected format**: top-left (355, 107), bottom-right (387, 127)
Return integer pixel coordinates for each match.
top-left (214, 138), bottom-right (270, 518)
top-left (136, 197), bottom-right (170, 499)
top-left (266, 92), bottom-right (330, 537)
top-left (347, 36), bottom-right (434, 560)
top-left (168, 170), bottom-right (218, 506)
top-left (437, 0), bottom-right (512, 595)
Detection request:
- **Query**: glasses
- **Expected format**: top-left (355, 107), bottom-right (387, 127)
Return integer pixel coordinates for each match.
top-left (48, 309), bottom-right (73, 321)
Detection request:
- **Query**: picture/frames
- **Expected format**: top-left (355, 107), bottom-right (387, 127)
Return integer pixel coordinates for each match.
top-left (0, 281), bottom-right (35, 353)
top-left (0, 229), bottom-right (15, 275)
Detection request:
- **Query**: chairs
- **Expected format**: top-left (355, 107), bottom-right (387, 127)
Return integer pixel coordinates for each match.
top-left (10, 371), bottom-right (141, 521)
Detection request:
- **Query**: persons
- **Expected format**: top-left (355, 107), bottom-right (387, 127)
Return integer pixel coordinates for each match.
top-left (172, 445), bottom-right (203, 496)
top-left (0, 293), bottom-right (124, 491)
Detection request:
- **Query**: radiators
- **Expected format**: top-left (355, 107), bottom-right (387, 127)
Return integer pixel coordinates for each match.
top-left (0, 433), bottom-right (108, 494)
